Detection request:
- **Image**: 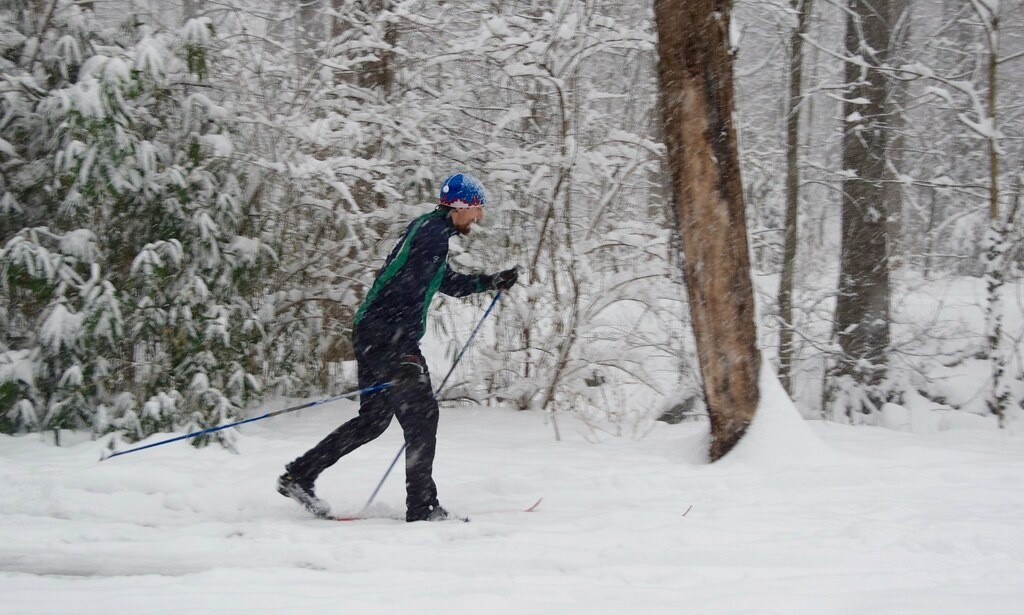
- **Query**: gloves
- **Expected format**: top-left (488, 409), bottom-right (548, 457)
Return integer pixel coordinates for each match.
top-left (482, 270), bottom-right (518, 290)
top-left (363, 343), bottom-right (397, 383)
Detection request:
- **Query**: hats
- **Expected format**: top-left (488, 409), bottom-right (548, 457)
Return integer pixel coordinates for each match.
top-left (440, 173), bottom-right (488, 208)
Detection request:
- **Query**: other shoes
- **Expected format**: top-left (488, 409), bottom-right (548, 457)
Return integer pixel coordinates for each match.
top-left (427, 507), bottom-right (470, 522)
top-left (276, 473), bottom-right (330, 515)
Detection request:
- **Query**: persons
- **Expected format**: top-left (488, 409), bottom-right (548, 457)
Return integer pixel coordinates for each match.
top-left (277, 171), bottom-right (519, 522)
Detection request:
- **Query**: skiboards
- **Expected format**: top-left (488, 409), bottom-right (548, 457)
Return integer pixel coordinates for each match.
top-left (320, 496), bottom-right (545, 523)
